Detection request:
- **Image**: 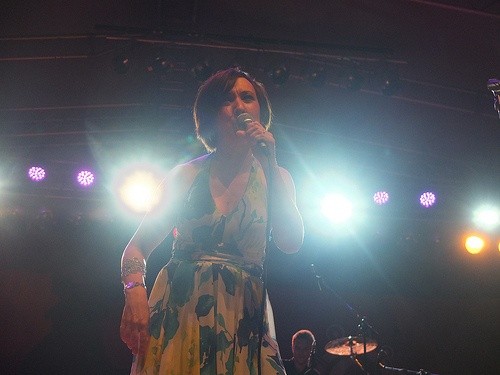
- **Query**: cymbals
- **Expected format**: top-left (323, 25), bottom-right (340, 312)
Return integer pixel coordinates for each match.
top-left (324, 334), bottom-right (379, 356)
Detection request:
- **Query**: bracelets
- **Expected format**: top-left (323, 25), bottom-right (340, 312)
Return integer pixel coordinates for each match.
top-left (121, 257), bottom-right (147, 282)
top-left (123, 282), bottom-right (148, 294)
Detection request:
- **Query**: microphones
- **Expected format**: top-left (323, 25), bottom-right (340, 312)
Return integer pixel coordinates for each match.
top-left (310, 264), bottom-right (322, 291)
top-left (236, 112), bottom-right (271, 157)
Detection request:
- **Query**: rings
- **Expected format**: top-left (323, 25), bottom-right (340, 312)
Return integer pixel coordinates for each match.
top-left (263, 129), bottom-right (266, 134)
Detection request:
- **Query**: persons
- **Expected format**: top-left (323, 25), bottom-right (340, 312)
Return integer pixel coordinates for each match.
top-left (120, 68), bottom-right (304, 375)
top-left (282, 330), bottom-right (321, 375)
